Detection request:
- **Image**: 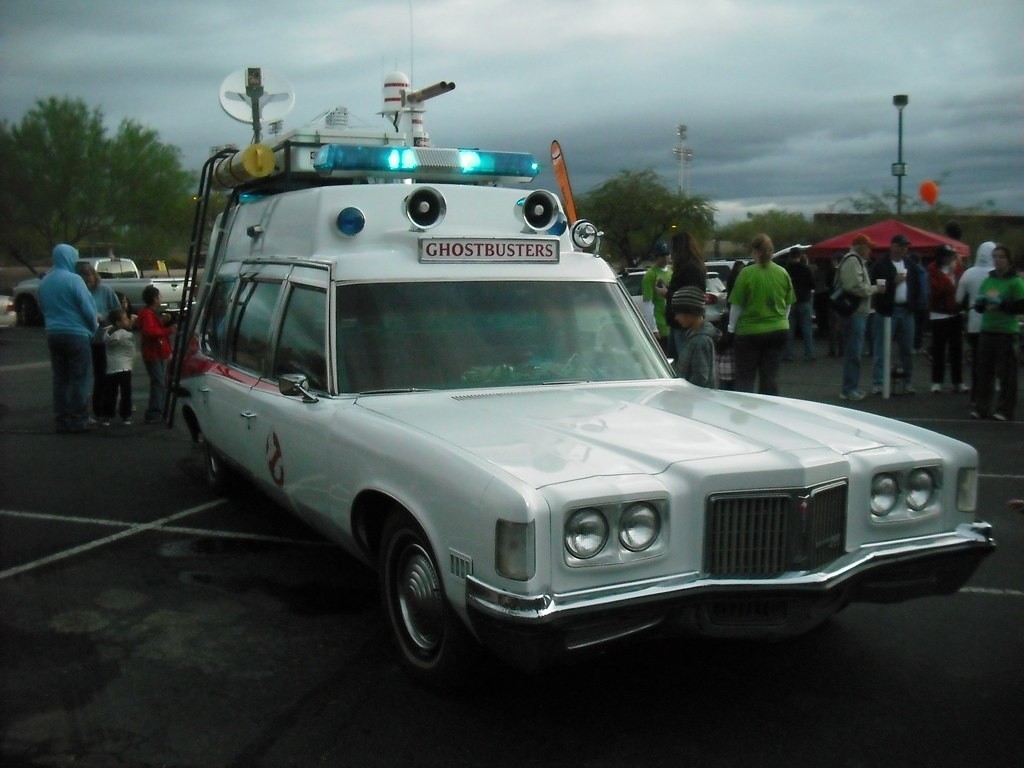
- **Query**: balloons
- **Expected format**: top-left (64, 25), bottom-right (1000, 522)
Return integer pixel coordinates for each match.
top-left (919, 180), bottom-right (938, 203)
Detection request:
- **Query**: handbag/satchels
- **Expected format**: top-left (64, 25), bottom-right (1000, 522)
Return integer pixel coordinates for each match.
top-left (828, 286), bottom-right (861, 317)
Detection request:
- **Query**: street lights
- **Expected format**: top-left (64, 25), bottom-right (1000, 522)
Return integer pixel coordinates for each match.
top-left (892, 95), bottom-right (909, 223)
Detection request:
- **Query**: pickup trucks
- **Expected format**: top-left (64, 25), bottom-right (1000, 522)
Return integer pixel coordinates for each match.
top-left (13, 257), bottom-right (196, 327)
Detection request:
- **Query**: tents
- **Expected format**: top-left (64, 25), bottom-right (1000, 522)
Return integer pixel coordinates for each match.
top-left (806, 219), bottom-right (970, 259)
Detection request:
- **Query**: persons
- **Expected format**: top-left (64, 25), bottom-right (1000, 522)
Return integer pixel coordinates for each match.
top-left (970, 246), bottom-right (1024, 420)
top-left (138, 285), bottom-right (177, 423)
top-left (909, 251), bottom-right (929, 355)
top-left (927, 244), bottom-right (970, 394)
top-left (38, 243), bottom-right (100, 432)
top-left (832, 234), bottom-right (886, 399)
top-left (955, 241), bottom-right (997, 399)
top-left (100, 309), bottom-right (133, 425)
top-left (784, 248), bottom-right (842, 362)
top-left (871, 234), bottom-right (920, 394)
top-left (725, 233), bottom-right (796, 397)
top-left (75, 263), bottom-right (121, 423)
top-left (654, 232), bottom-right (707, 359)
top-left (643, 241), bottom-right (673, 358)
top-left (669, 285), bottom-right (722, 389)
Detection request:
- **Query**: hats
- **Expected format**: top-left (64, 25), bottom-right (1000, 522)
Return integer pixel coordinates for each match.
top-left (654, 240), bottom-right (673, 255)
top-left (670, 285), bottom-right (708, 312)
top-left (852, 233), bottom-right (878, 248)
top-left (892, 235), bottom-right (910, 245)
top-left (933, 244), bottom-right (956, 264)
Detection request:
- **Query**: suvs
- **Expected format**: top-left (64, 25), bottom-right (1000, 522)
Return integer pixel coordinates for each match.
top-left (164, 69), bottom-right (1000, 680)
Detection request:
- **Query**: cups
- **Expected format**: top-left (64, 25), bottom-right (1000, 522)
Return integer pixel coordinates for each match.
top-left (877, 279), bottom-right (886, 287)
top-left (900, 268), bottom-right (907, 277)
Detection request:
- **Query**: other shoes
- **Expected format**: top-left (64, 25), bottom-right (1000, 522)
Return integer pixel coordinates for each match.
top-left (145, 415), bottom-right (161, 423)
top-left (123, 416), bottom-right (134, 425)
top-left (902, 382), bottom-right (915, 394)
top-left (56, 422), bottom-right (90, 434)
top-left (970, 412), bottom-right (980, 418)
top-left (131, 404), bottom-right (137, 411)
top-left (930, 382), bottom-right (944, 393)
top-left (839, 388), bottom-right (865, 400)
top-left (89, 413), bottom-right (99, 423)
top-left (102, 417), bottom-right (112, 427)
top-left (992, 412), bottom-right (1007, 420)
top-left (951, 383), bottom-right (969, 392)
top-left (871, 383), bottom-right (882, 394)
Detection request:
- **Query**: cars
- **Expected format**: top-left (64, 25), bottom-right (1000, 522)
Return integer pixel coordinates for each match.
top-left (616, 244), bottom-right (810, 346)
top-left (0, 295), bottom-right (17, 329)
